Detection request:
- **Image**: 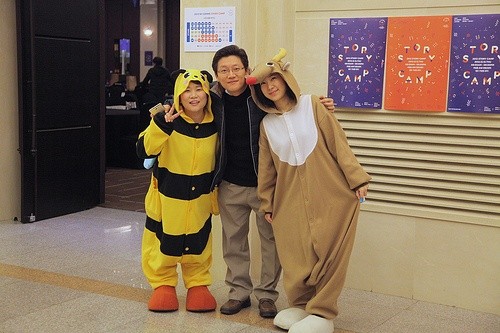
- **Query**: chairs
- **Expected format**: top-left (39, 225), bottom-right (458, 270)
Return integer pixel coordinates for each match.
top-left (105, 85), bottom-right (158, 169)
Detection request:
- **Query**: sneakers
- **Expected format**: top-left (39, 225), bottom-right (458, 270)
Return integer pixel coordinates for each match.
top-left (258, 299), bottom-right (278, 318)
top-left (219, 296), bottom-right (252, 315)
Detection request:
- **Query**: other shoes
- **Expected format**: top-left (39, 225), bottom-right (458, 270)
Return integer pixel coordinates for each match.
top-left (287, 315), bottom-right (335, 333)
top-left (187, 285), bottom-right (217, 311)
top-left (147, 285), bottom-right (179, 311)
top-left (273, 306), bottom-right (310, 329)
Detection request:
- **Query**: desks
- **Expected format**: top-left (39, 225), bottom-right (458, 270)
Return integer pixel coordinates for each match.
top-left (106, 106), bottom-right (141, 160)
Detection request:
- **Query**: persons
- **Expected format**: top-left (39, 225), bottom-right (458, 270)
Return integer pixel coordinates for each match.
top-left (141, 57), bottom-right (172, 105)
top-left (136, 69), bottom-right (221, 312)
top-left (246, 48), bottom-right (372, 333)
top-left (150, 45), bottom-right (335, 317)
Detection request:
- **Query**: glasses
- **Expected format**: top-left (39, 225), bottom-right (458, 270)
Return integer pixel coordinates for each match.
top-left (217, 66), bottom-right (245, 74)
